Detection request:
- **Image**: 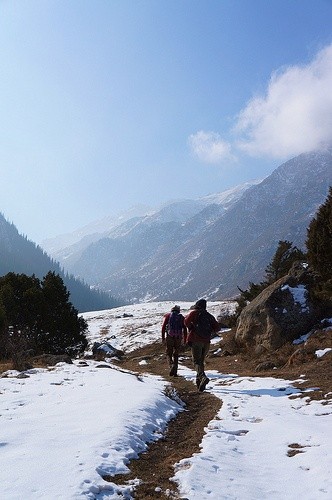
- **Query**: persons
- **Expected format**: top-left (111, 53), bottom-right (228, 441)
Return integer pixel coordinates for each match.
top-left (162, 304), bottom-right (188, 377)
top-left (183, 299), bottom-right (221, 392)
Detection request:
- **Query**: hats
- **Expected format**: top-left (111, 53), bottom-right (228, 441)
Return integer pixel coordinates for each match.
top-left (171, 305), bottom-right (180, 313)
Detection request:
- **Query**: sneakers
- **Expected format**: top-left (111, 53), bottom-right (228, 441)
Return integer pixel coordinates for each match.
top-left (199, 377), bottom-right (210, 392)
top-left (169, 363), bottom-right (178, 376)
top-left (196, 372), bottom-right (206, 389)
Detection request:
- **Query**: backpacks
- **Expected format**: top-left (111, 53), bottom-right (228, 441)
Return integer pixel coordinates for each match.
top-left (193, 310), bottom-right (213, 337)
top-left (166, 312), bottom-right (183, 338)
